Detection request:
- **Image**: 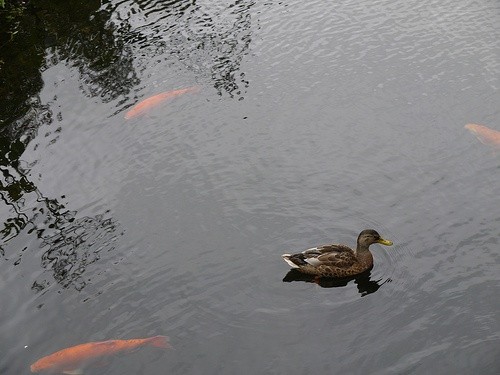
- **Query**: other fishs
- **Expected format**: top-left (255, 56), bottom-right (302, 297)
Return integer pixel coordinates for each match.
top-left (30, 335), bottom-right (171, 375)
top-left (124, 85), bottom-right (198, 120)
top-left (463, 124), bottom-right (500, 149)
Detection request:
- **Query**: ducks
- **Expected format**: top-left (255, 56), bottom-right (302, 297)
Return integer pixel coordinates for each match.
top-left (281, 229), bottom-right (393, 280)
top-left (282, 269), bottom-right (392, 298)
